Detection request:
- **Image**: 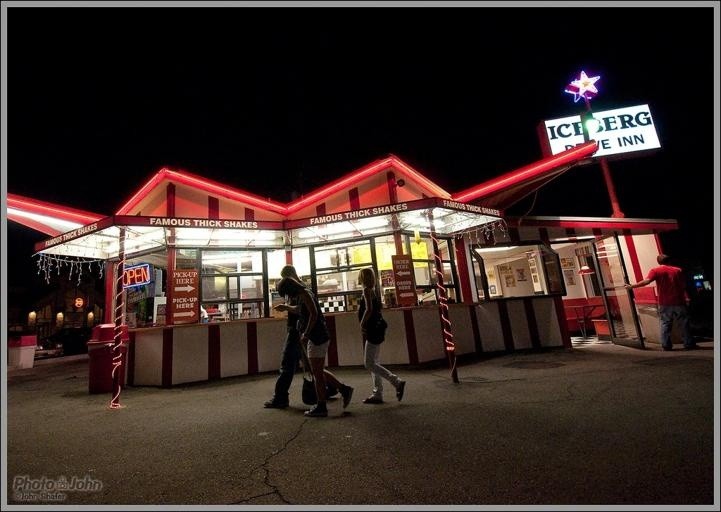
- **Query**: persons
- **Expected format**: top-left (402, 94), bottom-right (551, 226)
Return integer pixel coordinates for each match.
top-left (355, 267), bottom-right (407, 405)
top-left (277, 276), bottom-right (354, 418)
top-left (262, 263), bottom-right (340, 408)
top-left (623, 253), bottom-right (700, 351)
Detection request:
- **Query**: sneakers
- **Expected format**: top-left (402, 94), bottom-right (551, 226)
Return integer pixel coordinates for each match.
top-left (326, 385), bottom-right (339, 396)
top-left (304, 401), bottom-right (329, 417)
top-left (362, 395), bottom-right (385, 403)
top-left (264, 392), bottom-right (290, 408)
top-left (338, 383), bottom-right (354, 408)
top-left (396, 380), bottom-right (407, 401)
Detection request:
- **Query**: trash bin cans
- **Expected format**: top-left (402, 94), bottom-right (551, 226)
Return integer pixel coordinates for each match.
top-left (7, 335), bottom-right (38, 370)
top-left (87, 323), bottom-right (129, 395)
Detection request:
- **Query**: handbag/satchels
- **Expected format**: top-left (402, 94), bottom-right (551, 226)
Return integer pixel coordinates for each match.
top-left (361, 315), bottom-right (389, 345)
top-left (302, 376), bottom-right (318, 405)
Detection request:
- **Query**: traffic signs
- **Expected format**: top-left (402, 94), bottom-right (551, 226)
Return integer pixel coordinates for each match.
top-left (171, 270), bottom-right (200, 323)
top-left (391, 255), bottom-right (417, 305)
